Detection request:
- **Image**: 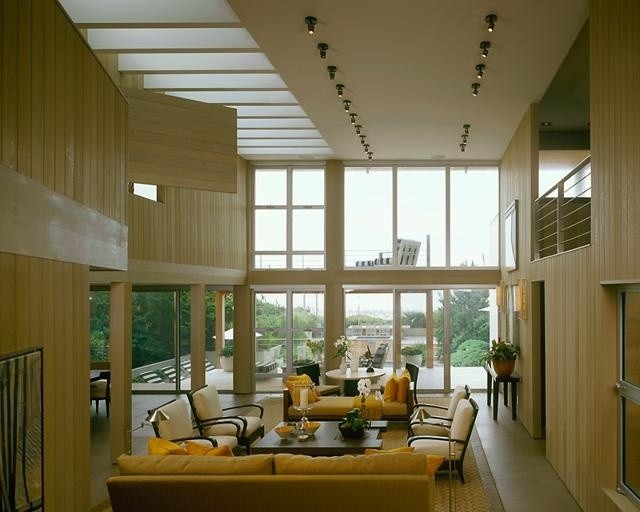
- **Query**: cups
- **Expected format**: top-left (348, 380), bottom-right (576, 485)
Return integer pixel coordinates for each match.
top-left (375, 391), bottom-right (382, 400)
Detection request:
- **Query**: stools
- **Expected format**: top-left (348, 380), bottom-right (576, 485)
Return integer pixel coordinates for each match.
top-left (356, 260), bottom-right (374, 267)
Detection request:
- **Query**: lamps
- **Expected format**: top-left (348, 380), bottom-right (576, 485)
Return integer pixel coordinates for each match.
top-left (304, 14), bottom-right (499, 162)
top-left (512, 278), bottom-right (529, 320)
top-left (495, 280), bottom-right (507, 313)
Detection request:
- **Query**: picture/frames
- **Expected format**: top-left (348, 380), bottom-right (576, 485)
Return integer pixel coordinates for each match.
top-left (503, 198), bottom-right (520, 273)
top-left (0, 345), bottom-right (45, 511)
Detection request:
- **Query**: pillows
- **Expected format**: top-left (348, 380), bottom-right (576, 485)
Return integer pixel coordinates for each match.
top-left (282, 369), bottom-right (411, 406)
top-left (117, 436), bottom-right (445, 474)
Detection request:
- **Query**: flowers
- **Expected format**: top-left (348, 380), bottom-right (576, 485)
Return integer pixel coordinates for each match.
top-left (332, 336), bottom-right (352, 360)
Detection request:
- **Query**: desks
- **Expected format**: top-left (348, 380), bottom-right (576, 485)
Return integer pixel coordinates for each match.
top-left (486, 360), bottom-right (521, 421)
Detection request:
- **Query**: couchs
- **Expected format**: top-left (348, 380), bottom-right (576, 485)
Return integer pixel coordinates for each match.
top-left (105, 474), bottom-right (435, 512)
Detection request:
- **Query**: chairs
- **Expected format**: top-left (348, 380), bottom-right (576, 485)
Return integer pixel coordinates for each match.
top-left (408, 384), bottom-right (472, 437)
top-left (407, 397), bottom-right (479, 484)
top-left (147, 399), bottom-right (241, 456)
top-left (185, 384), bottom-right (265, 455)
top-left (296, 362), bottom-right (341, 397)
top-left (359, 343), bottom-right (389, 368)
top-left (90, 370), bottom-right (110, 417)
top-left (374, 238), bottom-right (421, 267)
top-left (381, 364), bottom-right (419, 408)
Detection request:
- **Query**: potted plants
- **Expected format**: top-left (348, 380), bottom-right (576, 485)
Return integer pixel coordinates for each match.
top-left (401, 347), bottom-right (423, 367)
top-left (479, 338), bottom-right (521, 377)
top-left (219, 346), bottom-right (233, 372)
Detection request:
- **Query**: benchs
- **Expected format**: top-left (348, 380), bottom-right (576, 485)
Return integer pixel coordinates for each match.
top-left (283, 388), bottom-right (414, 422)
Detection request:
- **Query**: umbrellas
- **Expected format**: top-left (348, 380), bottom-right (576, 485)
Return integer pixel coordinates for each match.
top-left (211, 326), bottom-right (263, 341)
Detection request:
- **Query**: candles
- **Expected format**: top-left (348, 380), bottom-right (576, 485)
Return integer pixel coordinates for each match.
top-left (300, 389), bottom-right (308, 409)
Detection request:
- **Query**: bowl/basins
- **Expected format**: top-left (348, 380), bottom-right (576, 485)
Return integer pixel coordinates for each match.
top-left (303, 422), bottom-right (321, 436)
top-left (297, 435), bottom-right (308, 442)
top-left (274, 426), bottom-right (294, 439)
top-left (286, 422), bottom-right (303, 434)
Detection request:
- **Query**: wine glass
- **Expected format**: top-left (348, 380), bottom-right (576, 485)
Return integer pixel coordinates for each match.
top-left (293, 383), bottom-right (316, 426)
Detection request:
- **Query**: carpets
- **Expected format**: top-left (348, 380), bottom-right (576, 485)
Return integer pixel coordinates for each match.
top-left (90, 396), bottom-right (506, 512)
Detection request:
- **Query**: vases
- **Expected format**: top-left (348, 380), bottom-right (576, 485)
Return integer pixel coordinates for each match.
top-left (361, 397), bottom-right (367, 418)
top-left (339, 356), bottom-right (347, 374)
